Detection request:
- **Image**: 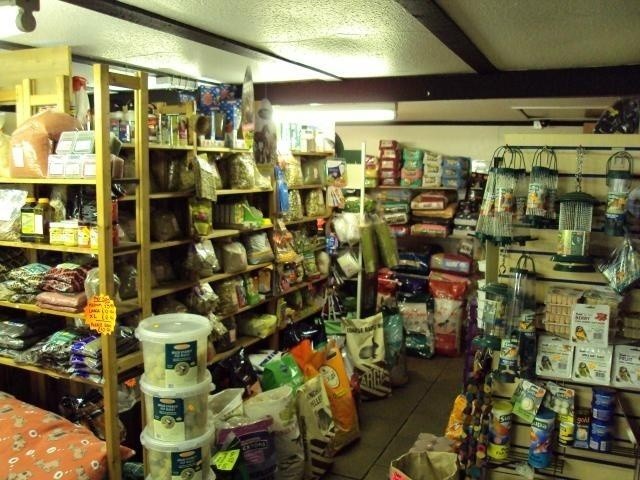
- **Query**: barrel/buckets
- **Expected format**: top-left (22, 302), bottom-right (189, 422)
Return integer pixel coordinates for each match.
top-left (139, 369), bottom-right (216, 440)
top-left (145, 468), bottom-right (216, 480)
top-left (138, 427), bottom-right (214, 480)
top-left (134, 313), bottom-right (212, 387)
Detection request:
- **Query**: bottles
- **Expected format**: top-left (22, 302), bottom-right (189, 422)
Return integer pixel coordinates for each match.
top-left (49, 219), bottom-right (120, 251)
top-left (109, 118), bottom-right (136, 146)
top-left (19, 197), bottom-right (56, 244)
